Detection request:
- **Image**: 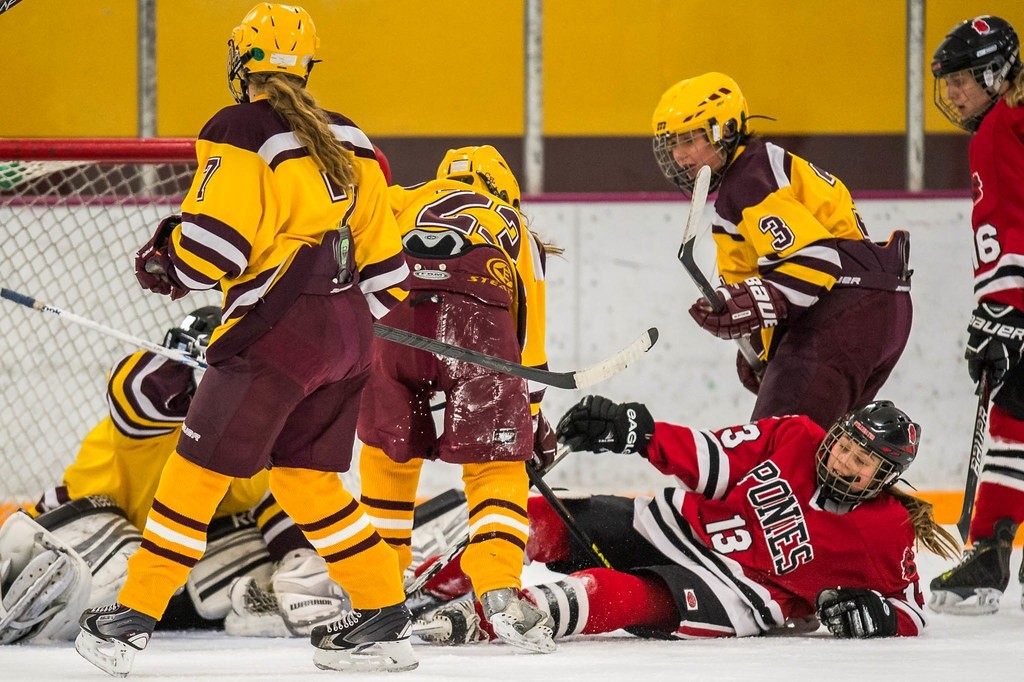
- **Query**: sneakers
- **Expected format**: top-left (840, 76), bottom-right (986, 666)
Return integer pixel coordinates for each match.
top-left (72, 604), bottom-right (158, 678)
top-left (928, 515), bottom-right (1020, 614)
top-left (412, 599), bottom-right (491, 646)
top-left (310, 601), bottom-right (419, 673)
top-left (482, 586), bottom-right (556, 654)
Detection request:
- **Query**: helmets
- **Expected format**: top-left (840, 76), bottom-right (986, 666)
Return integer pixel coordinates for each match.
top-left (931, 15), bottom-right (1022, 135)
top-left (652, 71), bottom-right (750, 197)
top-left (814, 399), bottom-right (922, 508)
top-left (436, 145), bottom-right (520, 210)
top-left (180, 305), bottom-right (226, 342)
top-left (226, 2), bottom-right (321, 102)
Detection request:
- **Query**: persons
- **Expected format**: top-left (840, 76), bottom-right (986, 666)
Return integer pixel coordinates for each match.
top-left (0, 305), bottom-right (351, 643)
top-left (399, 396), bottom-right (962, 647)
top-left (73, 2), bottom-right (418, 677)
top-left (359, 143), bottom-right (556, 656)
top-left (650, 70), bottom-right (913, 434)
top-left (925, 14), bottom-right (1024, 616)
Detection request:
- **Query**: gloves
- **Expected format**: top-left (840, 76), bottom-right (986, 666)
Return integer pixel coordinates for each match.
top-left (525, 412), bottom-right (556, 473)
top-left (965, 299), bottom-right (1024, 396)
top-left (736, 318), bottom-right (783, 394)
top-left (689, 277), bottom-right (786, 341)
top-left (135, 214), bottom-right (189, 302)
top-left (557, 396), bottom-right (657, 454)
top-left (819, 587), bottom-right (898, 640)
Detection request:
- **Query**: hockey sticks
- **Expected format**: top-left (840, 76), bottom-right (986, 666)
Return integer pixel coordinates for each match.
top-left (0, 287), bottom-right (209, 374)
top-left (921, 367), bottom-right (995, 556)
top-left (526, 461), bottom-right (614, 571)
top-left (371, 310), bottom-right (659, 393)
top-left (674, 163), bottom-right (767, 375)
